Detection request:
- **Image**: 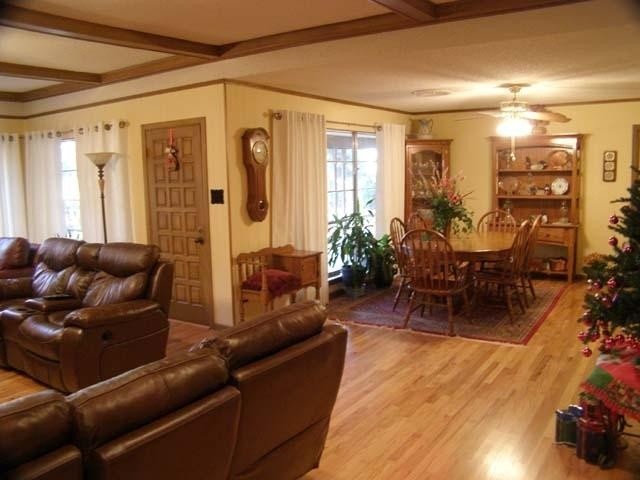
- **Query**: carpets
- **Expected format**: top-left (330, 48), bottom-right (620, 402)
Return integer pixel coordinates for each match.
top-left (327, 284), bottom-right (565, 346)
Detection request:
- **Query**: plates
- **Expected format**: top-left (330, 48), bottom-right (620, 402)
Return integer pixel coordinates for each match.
top-left (551, 177), bottom-right (569, 195)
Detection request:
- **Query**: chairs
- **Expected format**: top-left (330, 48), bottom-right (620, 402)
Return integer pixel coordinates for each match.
top-left (386, 208), bottom-right (544, 334)
top-left (237, 249), bottom-right (298, 323)
top-left (0, 235), bottom-right (174, 394)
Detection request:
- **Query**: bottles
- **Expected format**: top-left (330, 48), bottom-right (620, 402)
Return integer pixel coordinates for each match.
top-left (545, 184), bottom-right (550, 195)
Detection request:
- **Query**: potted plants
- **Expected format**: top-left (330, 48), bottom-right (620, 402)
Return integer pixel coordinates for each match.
top-left (327, 197), bottom-right (379, 290)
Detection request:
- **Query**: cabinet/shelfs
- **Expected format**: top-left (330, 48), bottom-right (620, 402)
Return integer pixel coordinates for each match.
top-left (404, 138), bottom-right (454, 227)
top-left (486, 133), bottom-right (586, 282)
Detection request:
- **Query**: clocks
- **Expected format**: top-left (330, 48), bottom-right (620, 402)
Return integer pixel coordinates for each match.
top-left (252, 140), bottom-right (268, 165)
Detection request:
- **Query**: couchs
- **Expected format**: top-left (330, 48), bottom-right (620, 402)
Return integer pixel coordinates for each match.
top-left (1, 300), bottom-right (349, 480)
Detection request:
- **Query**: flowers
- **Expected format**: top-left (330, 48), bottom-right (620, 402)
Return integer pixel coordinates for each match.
top-left (408, 159), bottom-right (480, 236)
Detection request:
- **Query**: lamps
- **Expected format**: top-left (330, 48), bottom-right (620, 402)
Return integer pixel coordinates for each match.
top-left (85, 151), bottom-right (116, 244)
